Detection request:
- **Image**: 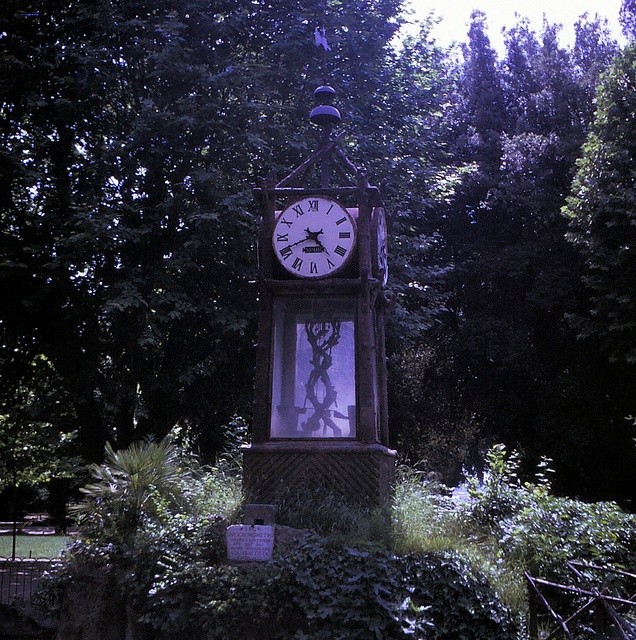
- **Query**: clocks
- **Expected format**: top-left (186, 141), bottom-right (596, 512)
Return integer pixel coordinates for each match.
top-left (270, 196), bottom-right (358, 280)
top-left (372, 203), bottom-right (388, 287)
